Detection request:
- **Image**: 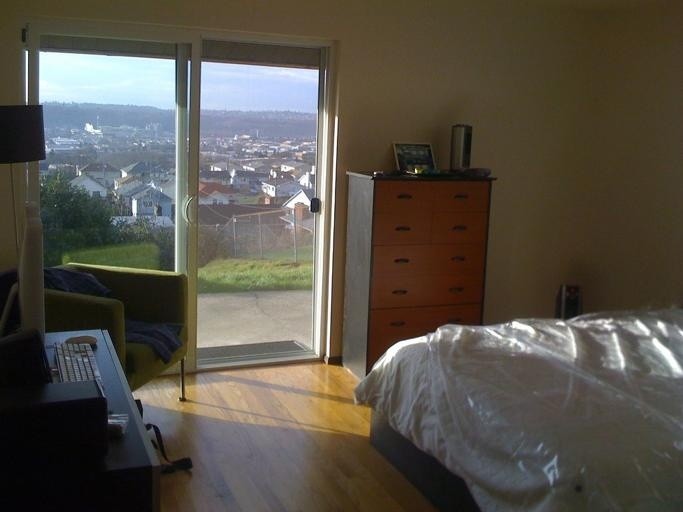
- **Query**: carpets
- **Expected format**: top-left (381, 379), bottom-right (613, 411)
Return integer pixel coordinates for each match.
top-left (196, 339), bottom-right (309, 365)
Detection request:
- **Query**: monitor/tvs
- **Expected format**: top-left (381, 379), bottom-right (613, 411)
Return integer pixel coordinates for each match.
top-left (0, 201), bottom-right (58, 375)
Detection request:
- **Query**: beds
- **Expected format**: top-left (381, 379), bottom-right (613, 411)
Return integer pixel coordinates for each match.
top-left (370, 305), bottom-right (683, 511)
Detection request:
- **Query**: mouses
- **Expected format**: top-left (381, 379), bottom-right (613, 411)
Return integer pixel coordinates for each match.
top-left (64, 336), bottom-right (96, 346)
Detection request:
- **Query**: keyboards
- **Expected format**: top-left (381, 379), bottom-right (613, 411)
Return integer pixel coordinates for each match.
top-left (52, 342), bottom-right (101, 383)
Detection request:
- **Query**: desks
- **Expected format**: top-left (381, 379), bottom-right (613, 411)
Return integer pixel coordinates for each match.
top-left (1, 329), bottom-right (161, 511)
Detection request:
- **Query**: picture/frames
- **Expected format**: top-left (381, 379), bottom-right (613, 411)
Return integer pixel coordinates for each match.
top-left (391, 141), bottom-right (436, 172)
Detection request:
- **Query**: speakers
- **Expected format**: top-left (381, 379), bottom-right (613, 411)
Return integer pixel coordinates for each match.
top-left (0, 329), bottom-right (51, 399)
top-left (0, 380), bottom-right (112, 470)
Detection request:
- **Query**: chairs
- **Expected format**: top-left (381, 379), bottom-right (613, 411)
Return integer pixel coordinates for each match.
top-left (1, 262), bottom-right (187, 403)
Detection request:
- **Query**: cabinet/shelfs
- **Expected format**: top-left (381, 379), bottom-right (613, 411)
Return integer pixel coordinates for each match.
top-left (341, 168), bottom-right (498, 384)
